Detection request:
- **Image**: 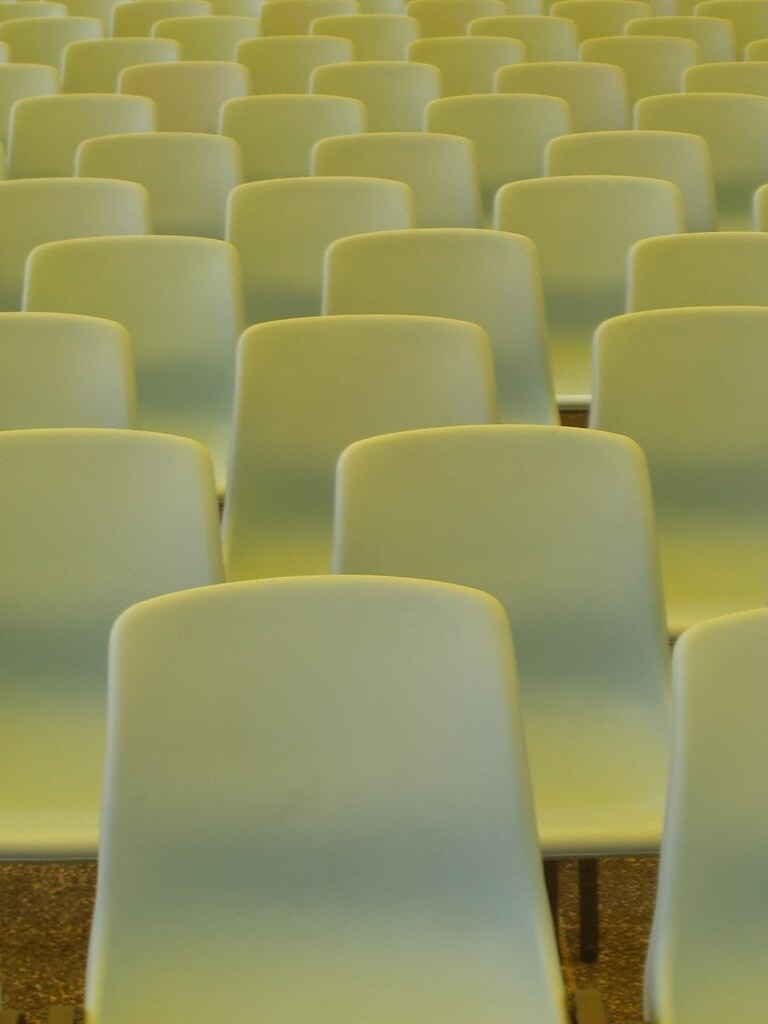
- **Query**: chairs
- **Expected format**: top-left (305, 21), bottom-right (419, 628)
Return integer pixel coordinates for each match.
top-left (0, 0), bottom-right (767, 1022)
